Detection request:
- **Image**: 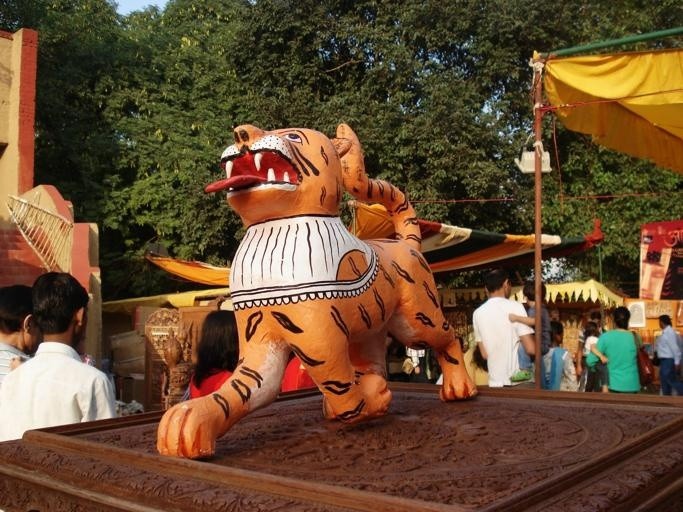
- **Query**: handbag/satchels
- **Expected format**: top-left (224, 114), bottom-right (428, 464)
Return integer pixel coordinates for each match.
top-left (637, 347), bottom-right (653, 386)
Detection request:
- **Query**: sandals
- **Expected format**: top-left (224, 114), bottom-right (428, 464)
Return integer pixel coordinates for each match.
top-left (511, 371), bottom-right (530, 382)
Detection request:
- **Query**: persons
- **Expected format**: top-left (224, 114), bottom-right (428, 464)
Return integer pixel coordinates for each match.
top-left (188, 309), bottom-right (239, 402)
top-left (455, 267), bottom-right (682, 396)
top-left (0, 284), bottom-right (41, 387)
top-left (0, 271), bottom-right (120, 445)
